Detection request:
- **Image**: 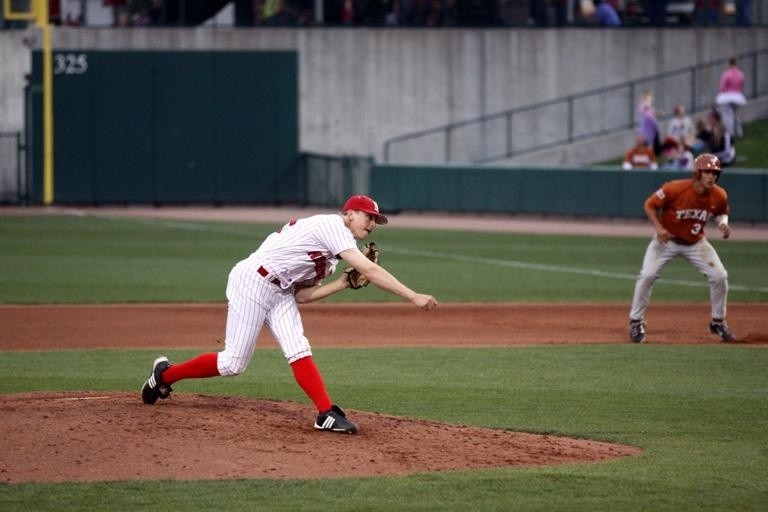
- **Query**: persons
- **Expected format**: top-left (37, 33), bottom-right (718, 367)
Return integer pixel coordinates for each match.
top-left (628, 153), bottom-right (737, 344)
top-left (716, 56), bottom-right (747, 139)
top-left (623, 91), bottom-right (735, 171)
top-left (140, 194), bottom-right (439, 435)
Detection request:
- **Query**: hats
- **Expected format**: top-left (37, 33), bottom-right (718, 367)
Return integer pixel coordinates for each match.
top-left (342, 194), bottom-right (389, 225)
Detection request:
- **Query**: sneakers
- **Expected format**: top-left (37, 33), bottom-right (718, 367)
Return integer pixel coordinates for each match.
top-left (313, 404), bottom-right (357, 432)
top-left (711, 322), bottom-right (738, 342)
top-left (141, 357), bottom-right (174, 407)
top-left (628, 319), bottom-right (647, 343)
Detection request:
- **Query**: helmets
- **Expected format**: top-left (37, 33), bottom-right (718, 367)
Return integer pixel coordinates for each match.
top-left (692, 154), bottom-right (723, 181)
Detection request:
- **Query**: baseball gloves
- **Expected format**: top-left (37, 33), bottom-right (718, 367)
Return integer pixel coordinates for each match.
top-left (345, 244), bottom-right (379, 289)
